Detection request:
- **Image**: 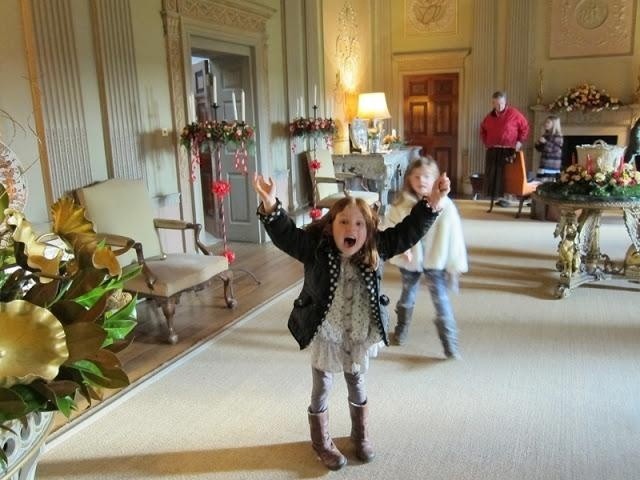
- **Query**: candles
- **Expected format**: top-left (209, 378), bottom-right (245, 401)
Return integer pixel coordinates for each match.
top-left (297, 99), bottom-right (299, 113)
top-left (241, 90), bottom-right (246, 122)
top-left (232, 92), bottom-right (237, 120)
top-left (314, 85), bottom-right (317, 105)
top-left (212, 75), bottom-right (217, 102)
top-left (300, 98), bottom-right (303, 116)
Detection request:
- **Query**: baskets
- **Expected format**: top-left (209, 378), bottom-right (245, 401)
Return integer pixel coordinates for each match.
top-left (576, 139), bottom-right (627, 172)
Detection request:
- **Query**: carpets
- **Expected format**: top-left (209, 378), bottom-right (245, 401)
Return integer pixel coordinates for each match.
top-left (33, 216), bottom-right (640, 480)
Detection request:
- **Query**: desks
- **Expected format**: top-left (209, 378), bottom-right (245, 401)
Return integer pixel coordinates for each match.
top-left (334, 144), bottom-right (424, 214)
top-left (531, 185), bottom-right (639, 299)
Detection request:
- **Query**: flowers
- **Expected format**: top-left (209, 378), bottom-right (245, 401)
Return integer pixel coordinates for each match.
top-left (549, 163), bottom-right (640, 201)
top-left (549, 84), bottom-right (620, 111)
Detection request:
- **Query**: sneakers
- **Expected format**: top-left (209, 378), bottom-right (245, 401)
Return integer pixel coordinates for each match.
top-left (495, 200), bottom-right (509, 206)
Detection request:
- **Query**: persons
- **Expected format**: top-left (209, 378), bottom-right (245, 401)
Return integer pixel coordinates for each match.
top-left (622, 117), bottom-right (640, 170)
top-left (535, 114), bottom-right (562, 179)
top-left (374, 157), bottom-right (468, 361)
top-left (249, 171), bottom-right (450, 472)
top-left (480, 92), bottom-right (530, 207)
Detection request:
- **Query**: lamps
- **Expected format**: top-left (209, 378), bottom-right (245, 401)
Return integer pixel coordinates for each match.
top-left (358, 92), bottom-right (391, 152)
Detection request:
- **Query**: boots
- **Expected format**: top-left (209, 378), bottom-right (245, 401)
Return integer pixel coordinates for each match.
top-left (348, 396), bottom-right (375, 462)
top-left (394, 301), bottom-right (414, 344)
top-left (307, 407), bottom-right (347, 470)
top-left (435, 316), bottom-right (456, 356)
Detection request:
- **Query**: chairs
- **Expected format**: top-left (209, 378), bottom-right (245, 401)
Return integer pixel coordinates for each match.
top-left (486, 147), bottom-right (542, 219)
top-left (305, 148), bottom-right (381, 222)
top-left (72, 177), bottom-right (237, 344)
